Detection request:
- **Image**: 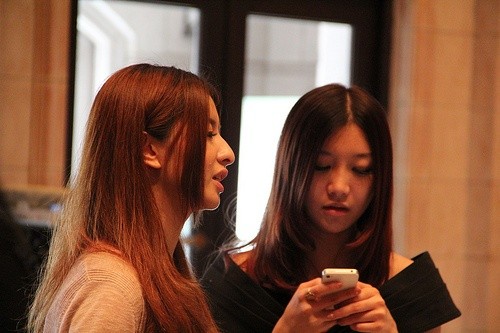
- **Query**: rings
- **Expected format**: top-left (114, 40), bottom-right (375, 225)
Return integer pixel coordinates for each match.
top-left (305, 288), bottom-right (319, 302)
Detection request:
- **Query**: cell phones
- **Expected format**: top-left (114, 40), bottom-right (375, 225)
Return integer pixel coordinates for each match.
top-left (321, 268), bottom-right (359, 310)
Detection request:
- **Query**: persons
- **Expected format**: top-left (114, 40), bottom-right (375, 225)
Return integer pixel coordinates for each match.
top-left (200, 83), bottom-right (462, 333)
top-left (28, 62), bottom-right (235, 333)
